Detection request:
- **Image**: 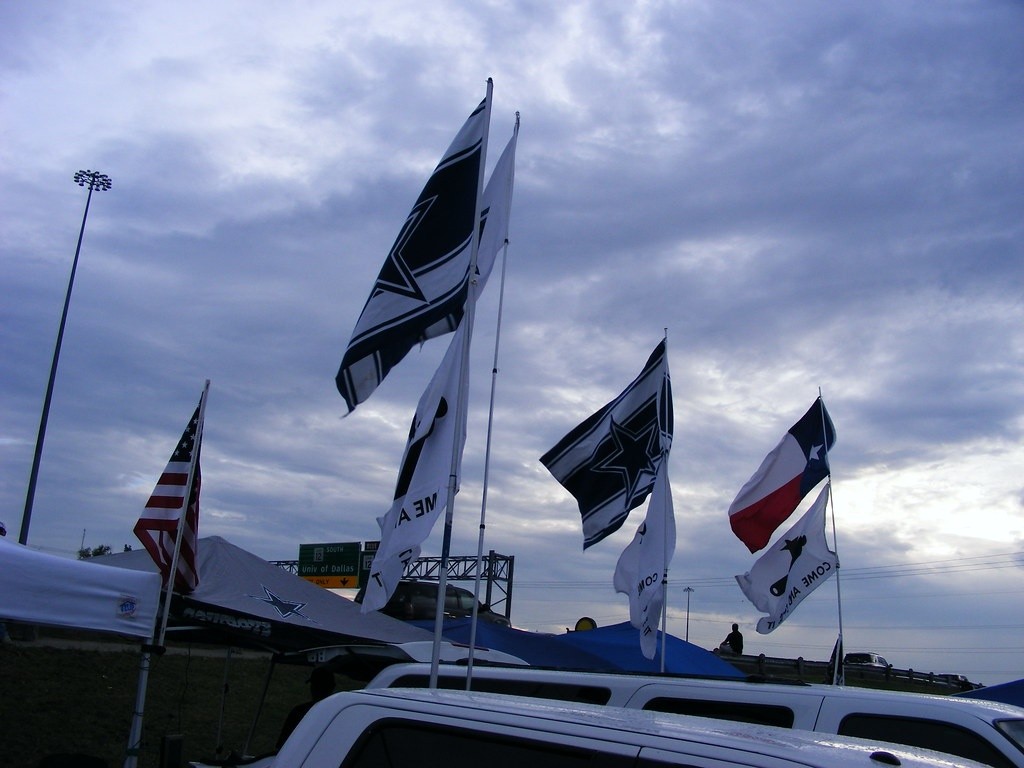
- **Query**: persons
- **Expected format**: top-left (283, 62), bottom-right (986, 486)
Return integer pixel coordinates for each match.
top-left (720, 623), bottom-right (743, 654)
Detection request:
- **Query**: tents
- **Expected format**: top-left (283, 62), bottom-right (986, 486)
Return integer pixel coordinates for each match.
top-left (0, 535), bottom-right (747, 768)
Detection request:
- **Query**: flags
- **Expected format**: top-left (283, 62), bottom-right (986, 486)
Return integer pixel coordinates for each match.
top-left (539, 337), bottom-right (677, 660)
top-left (727, 396), bottom-right (841, 634)
top-left (335, 97), bottom-right (520, 614)
top-left (132, 391), bottom-right (205, 594)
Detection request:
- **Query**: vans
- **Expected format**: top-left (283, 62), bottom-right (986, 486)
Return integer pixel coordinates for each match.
top-left (273, 659), bottom-right (1023, 766)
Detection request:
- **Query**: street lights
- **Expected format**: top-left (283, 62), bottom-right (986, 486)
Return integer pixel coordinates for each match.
top-left (17, 169), bottom-right (113, 547)
